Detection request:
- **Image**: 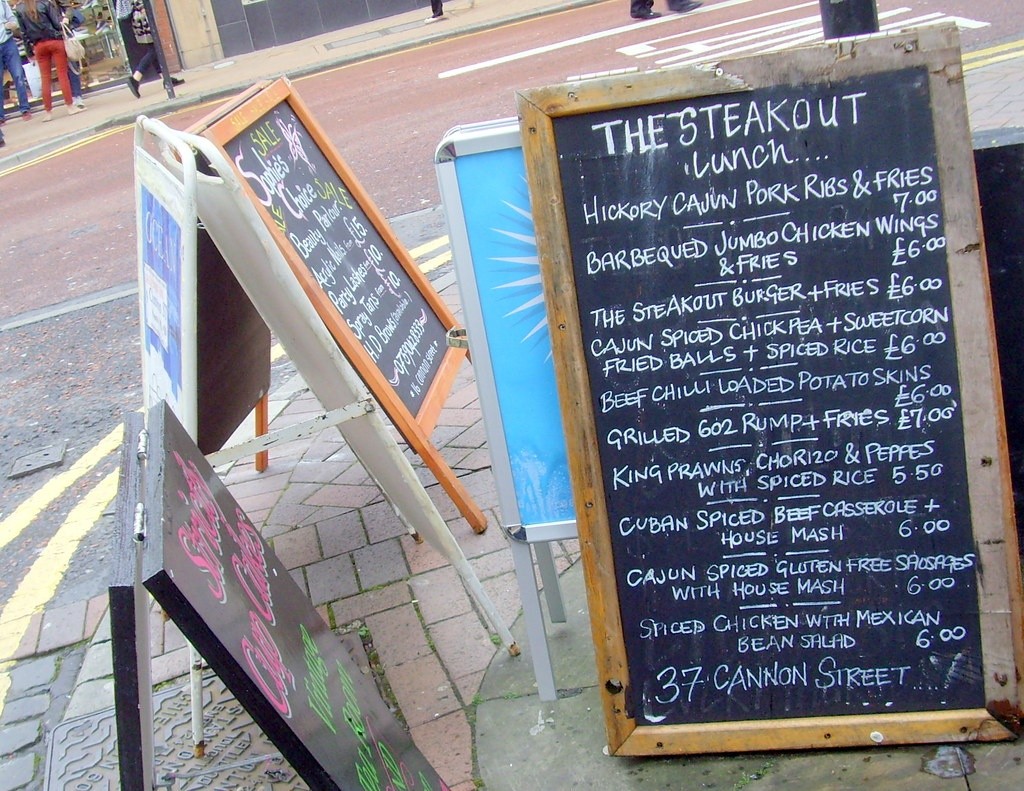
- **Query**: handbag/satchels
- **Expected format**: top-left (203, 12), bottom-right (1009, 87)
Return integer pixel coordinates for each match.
top-left (22, 61), bottom-right (53, 98)
top-left (60, 22), bottom-right (85, 74)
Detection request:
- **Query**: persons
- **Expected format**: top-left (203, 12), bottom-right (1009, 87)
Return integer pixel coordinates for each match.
top-left (629, 0), bottom-right (704, 21)
top-left (115, 0), bottom-right (185, 100)
top-left (46, 0), bottom-right (85, 110)
top-left (422, 0), bottom-right (448, 25)
top-left (15, 1), bottom-right (84, 122)
top-left (0, 0), bottom-right (33, 127)
top-left (0, 129), bottom-right (8, 148)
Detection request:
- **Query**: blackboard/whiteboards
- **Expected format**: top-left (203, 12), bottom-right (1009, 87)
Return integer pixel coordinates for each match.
top-left (970, 126), bottom-right (1023, 559)
top-left (174, 74), bottom-right (489, 538)
top-left (105, 401), bottom-right (451, 791)
top-left (511, 17), bottom-right (1023, 758)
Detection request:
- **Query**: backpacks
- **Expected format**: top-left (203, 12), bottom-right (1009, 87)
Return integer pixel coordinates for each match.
top-left (116, 0), bottom-right (134, 20)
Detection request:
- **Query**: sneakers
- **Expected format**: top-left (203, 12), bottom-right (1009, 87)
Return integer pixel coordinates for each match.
top-left (22, 112), bottom-right (32, 121)
top-left (0, 139), bottom-right (5, 148)
top-left (125, 77), bottom-right (140, 98)
top-left (67, 108), bottom-right (88, 116)
top-left (42, 115), bottom-right (52, 121)
top-left (676, 1), bottom-right (703, 13)
top-left (423, 14), bottom-right (447, 23)
top-left (0, 120), bottom-right (6, 126)
top-left (634, 10), bottom-right (661, 19)
top-left (76, 97), bottom-right (84, 106)
top-left (163, 77), bottom-right (185, 89)
